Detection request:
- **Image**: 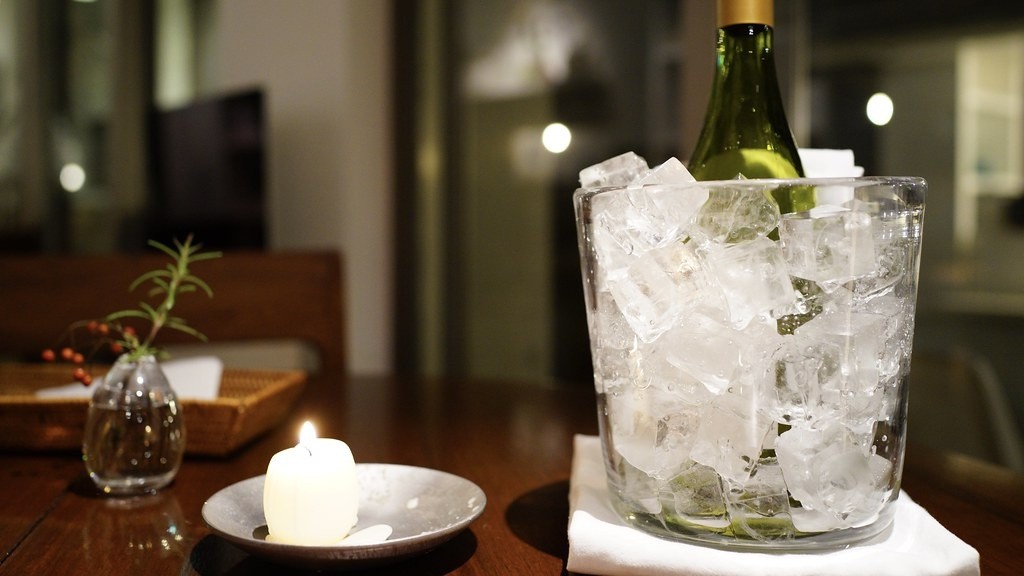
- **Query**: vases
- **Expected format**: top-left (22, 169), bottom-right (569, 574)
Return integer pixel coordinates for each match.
top-left (83, 354), bottom-right (186, 493)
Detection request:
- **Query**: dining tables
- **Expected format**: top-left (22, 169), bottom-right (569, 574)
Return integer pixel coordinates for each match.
top-left (0, 356), bottom-right (1024, 576)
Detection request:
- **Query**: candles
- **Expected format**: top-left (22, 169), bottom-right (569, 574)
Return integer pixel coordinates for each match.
top-left (262, 421), bottom-right (360, 546)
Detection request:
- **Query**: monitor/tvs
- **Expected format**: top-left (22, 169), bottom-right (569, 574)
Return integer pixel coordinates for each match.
top-left (143, 88), bottom-right (268, 253)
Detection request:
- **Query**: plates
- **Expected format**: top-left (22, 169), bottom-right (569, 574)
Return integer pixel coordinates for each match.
top-left (199, 461), bottom-right (488, 560)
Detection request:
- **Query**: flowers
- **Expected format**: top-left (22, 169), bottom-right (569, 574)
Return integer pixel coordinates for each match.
top-left (42, 234), bottom-right (222, 478)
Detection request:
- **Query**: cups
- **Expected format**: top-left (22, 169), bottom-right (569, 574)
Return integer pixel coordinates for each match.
top-left (571, 174), bottom-right (930, 556)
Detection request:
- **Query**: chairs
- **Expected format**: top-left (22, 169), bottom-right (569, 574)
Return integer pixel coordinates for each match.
top-left (0, 249), bottom-right (344, 374)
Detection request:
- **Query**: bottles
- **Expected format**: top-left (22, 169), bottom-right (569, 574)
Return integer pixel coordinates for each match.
top-left (685, 0), bottom-right (826, 509)
